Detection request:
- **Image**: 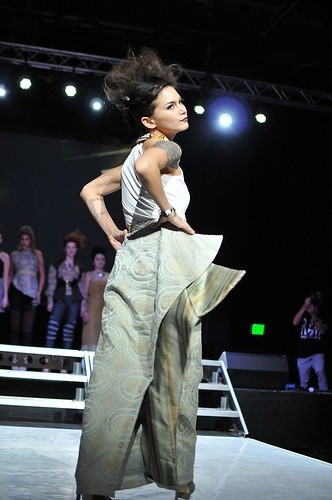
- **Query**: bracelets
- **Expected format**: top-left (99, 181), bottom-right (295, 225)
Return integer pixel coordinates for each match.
top-left (163, 207), bottom-right (175, 217)
top-left (313, 318), bottom-right (319, 323)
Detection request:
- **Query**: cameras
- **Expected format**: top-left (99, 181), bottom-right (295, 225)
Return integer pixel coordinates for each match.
top-left (310, 292), bottom-right (320, 307)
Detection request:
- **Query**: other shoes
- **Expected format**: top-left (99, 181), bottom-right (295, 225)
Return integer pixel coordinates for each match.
top-left (61, 369), bottom-right (68, 374)
top-left (20, 365), bottom-right (27, 371)
top-left (75, 491), bottom-right (113, 500)
top-left (11, 366), bottom-right (19, 370)
top-left (42, 368), bottom-right (49, 372)
top-left (175, 481), bottom-right (195, 500)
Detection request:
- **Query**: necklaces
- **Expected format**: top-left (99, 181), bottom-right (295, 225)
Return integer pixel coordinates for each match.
top-left (150, 132), bottom-right (164, 140)
top-left (65, 259), bottom-right (71, 268)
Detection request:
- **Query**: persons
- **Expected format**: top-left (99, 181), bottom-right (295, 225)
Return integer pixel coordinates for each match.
top-left (0, 225), bottom-right (332, 391)
top-left (73, 48), bottom-right (247, 500)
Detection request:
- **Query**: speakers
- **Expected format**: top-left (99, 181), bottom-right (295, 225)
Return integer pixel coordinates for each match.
top-left (219, 351), bottom-right (289, 390)
